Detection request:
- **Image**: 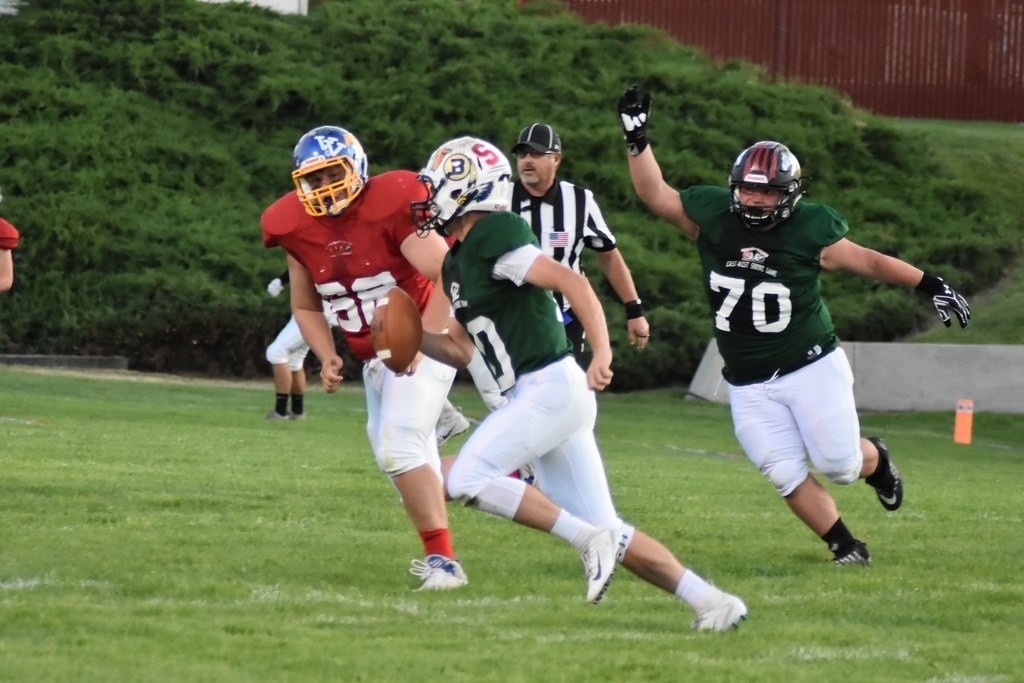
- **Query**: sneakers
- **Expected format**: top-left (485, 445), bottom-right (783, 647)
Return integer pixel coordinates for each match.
top-left (866, 437), bottom-right (903, 511)
top-left (519, 461), bottom-right (537, 486)
top-left (579, 527), bottom-right (629, 605)
top-left (689, 580), bottom-right (748, 633)
top-left (832, 540), bottom-right (872, 566)
top-left (409, 554), bottom-right (468, 592)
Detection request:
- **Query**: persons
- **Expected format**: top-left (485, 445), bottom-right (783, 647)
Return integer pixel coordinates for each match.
top-left (262, 122), bottom-right (748, 639)
top-left (0, 195), bottom-right (20, 294)
top-left (267, 269), bottom-right (339, 422)
top-left (617, 83), bottom-right (972, 569)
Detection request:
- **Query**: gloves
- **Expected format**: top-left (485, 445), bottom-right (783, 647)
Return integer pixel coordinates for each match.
top-left (617, 84), bottom-right (652, 156)
top-left (916, 271), bottom-right (972, 328)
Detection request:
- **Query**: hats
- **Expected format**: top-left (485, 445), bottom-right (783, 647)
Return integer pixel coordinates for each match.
top-left (510, 123), bottom-right (562, 154)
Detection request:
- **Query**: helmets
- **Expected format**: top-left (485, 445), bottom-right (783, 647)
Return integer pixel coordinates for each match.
top-left (727, 140), bottom-right (813, 233)
top-left (411, 135), bottom-right (515, 241)
top-left (292, 126), bottom-right (369, 217)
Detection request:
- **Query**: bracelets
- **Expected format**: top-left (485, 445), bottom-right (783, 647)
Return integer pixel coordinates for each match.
top-left (623, 299), bottom-right (646, 319)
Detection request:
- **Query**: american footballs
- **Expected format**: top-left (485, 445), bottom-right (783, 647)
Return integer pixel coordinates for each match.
top-left (370, 284), bottom-right (422, 374)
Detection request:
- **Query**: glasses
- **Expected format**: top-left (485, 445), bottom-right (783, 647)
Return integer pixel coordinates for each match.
top-left (517, 147), bottom-right (556, 158)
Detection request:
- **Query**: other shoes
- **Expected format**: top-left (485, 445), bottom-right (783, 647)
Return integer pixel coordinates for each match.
top-left (265, 408), bottom-right (307, 421)
top-left (434, 406), bottom-right (471, 447)
